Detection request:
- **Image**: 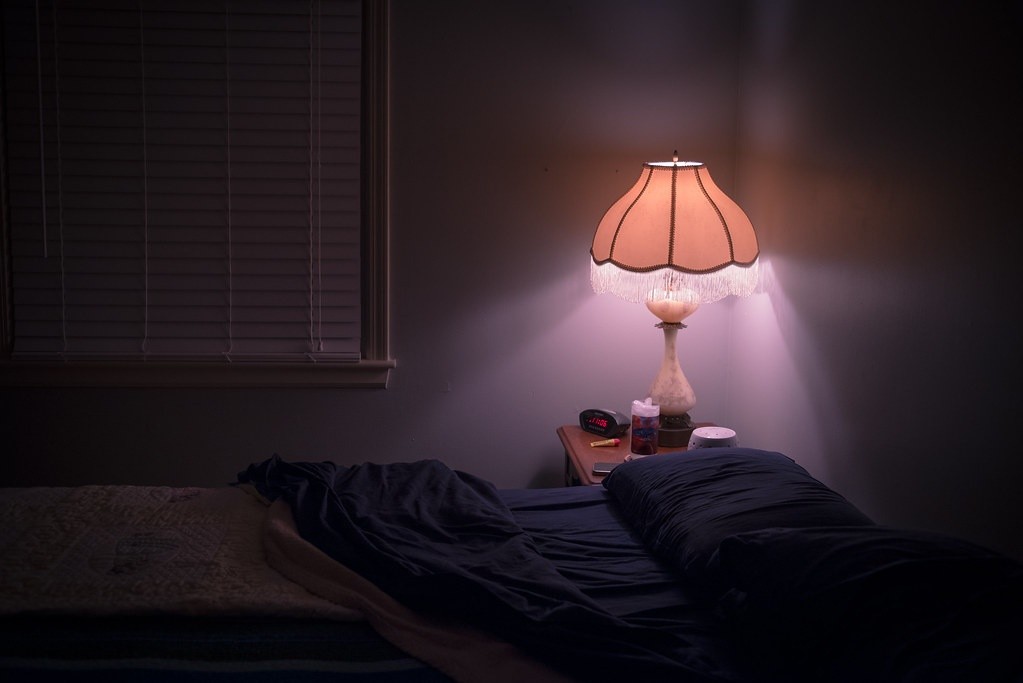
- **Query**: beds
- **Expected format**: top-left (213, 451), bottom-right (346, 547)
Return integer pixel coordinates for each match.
top-left (1, 437), bottom-right (1021, 683)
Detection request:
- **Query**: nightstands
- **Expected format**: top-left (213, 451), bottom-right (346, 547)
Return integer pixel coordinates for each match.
top-left (557, 417), bottom-right (729, 487)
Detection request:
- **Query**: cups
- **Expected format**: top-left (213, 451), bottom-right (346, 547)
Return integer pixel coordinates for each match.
top-left (630, 400), bottom-right (660, 457)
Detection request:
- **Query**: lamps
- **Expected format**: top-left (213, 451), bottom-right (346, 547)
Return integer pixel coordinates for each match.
top-left (588, 150), bottom-right (761, 443)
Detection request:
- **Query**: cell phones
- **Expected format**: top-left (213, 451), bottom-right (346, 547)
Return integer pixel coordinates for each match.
top-left (592, 462), bottom-right (624, 476)
